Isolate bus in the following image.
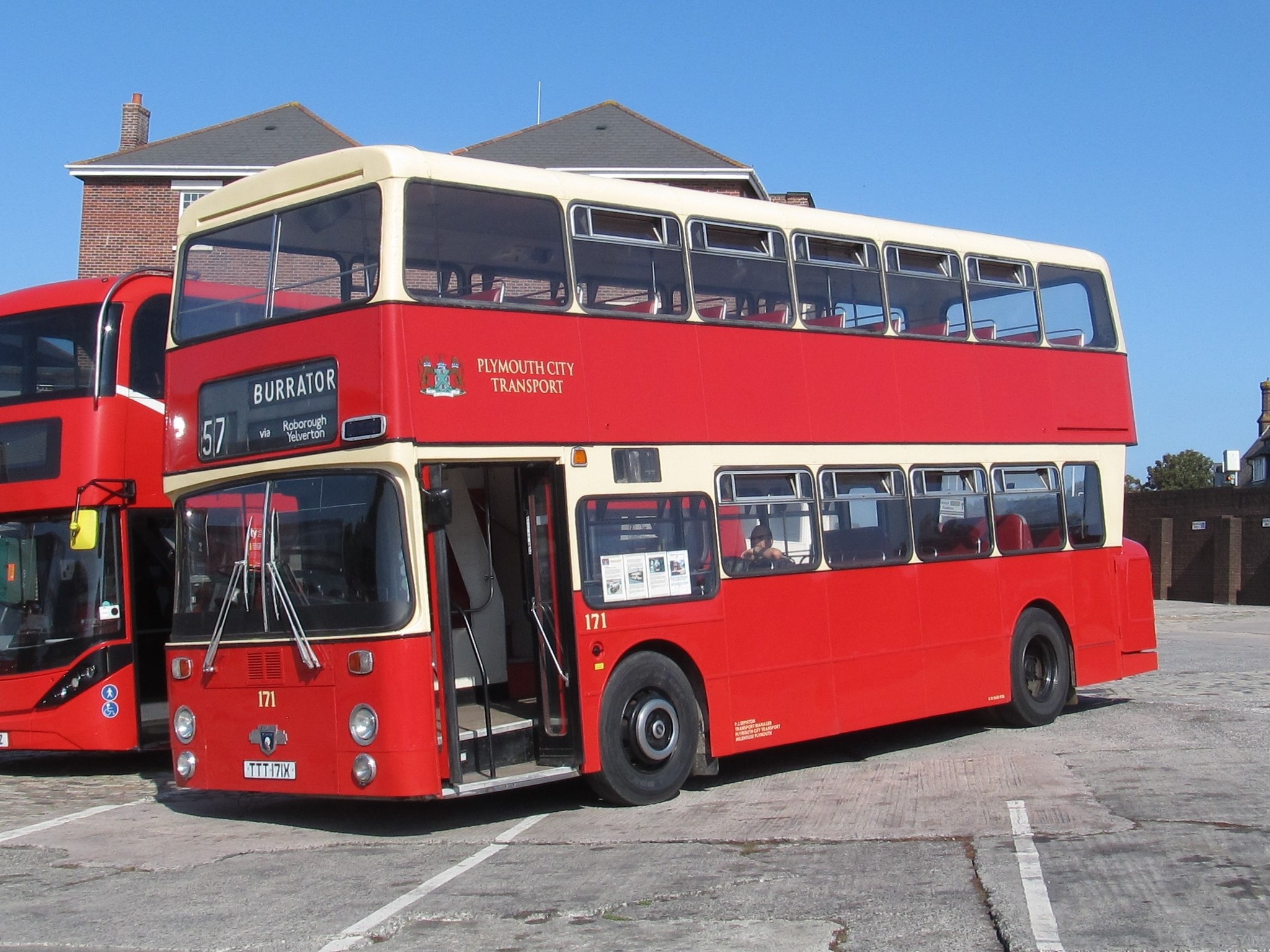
[0,263,341,752]
[160,143,1162,806]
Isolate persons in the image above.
[739,524,785,563]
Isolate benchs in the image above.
[435,277,1086,345]
[823,514,1062,557]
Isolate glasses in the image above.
[749,534,771,542]
